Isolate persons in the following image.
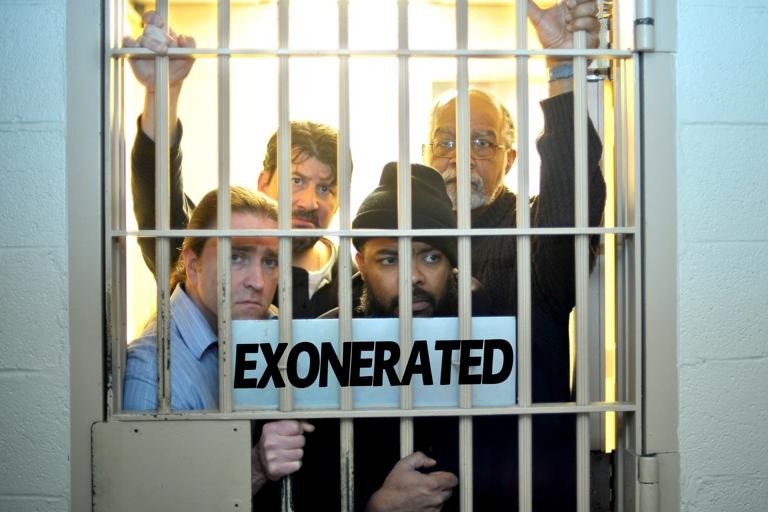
[422,0,605,399]
[122,9,361,319]
[293,161,577,512]
[123,185,315,494]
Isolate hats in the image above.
[352,162,458,267]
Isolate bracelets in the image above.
[548,63,572,82]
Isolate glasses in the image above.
[430,138,505,157]
